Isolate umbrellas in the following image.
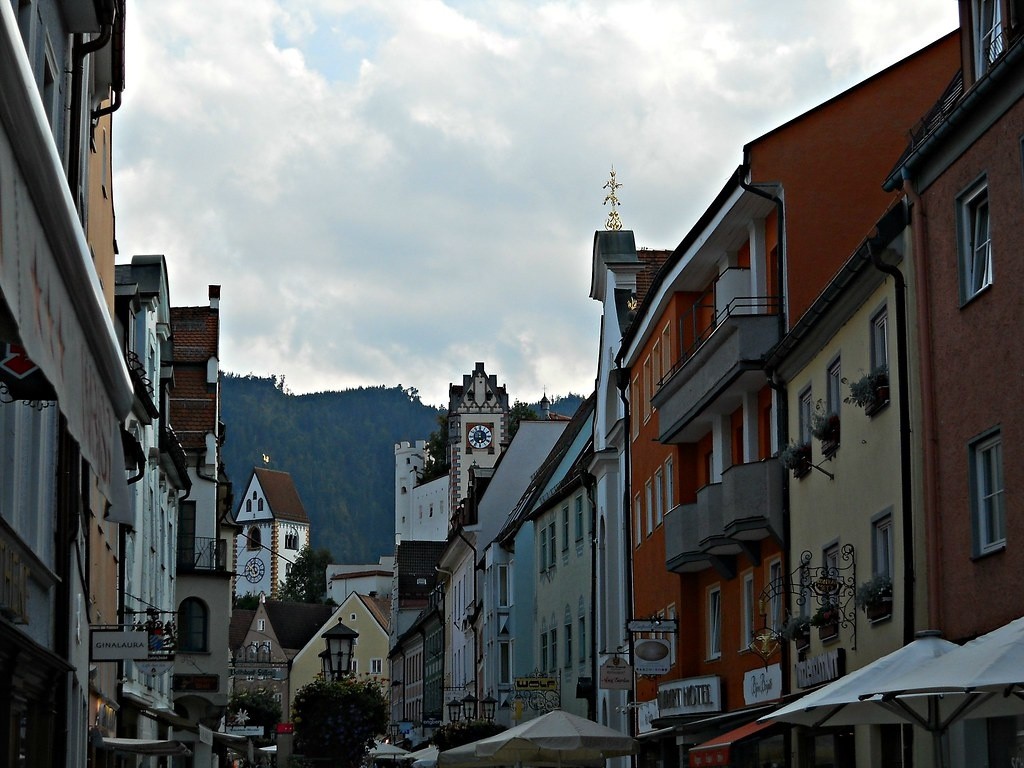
[258,745,277,754]
[758,616,1024,768]
[367,709,641,768]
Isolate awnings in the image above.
[147,707,254,762]
[688,719,780,768]
[102,737,193,759]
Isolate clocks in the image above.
[468,425,492,449]
[245,558,265,583]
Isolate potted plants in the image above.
[803,411,841,455]
[843,363,888,415]
[784,615,811,650]
[854,575,893,619]
[772,438,812,477]
[811,602,840,639]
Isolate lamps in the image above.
[749,543,857,672]
